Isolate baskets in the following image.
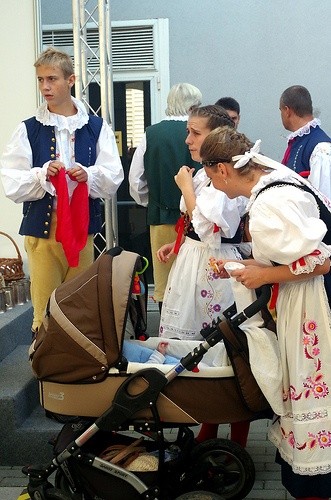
[0,230,24,280]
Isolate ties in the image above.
[281,138,294,164]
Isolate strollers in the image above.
[21,247,284,500]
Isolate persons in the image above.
[0,47,125,423]
[121,82,331,500]
